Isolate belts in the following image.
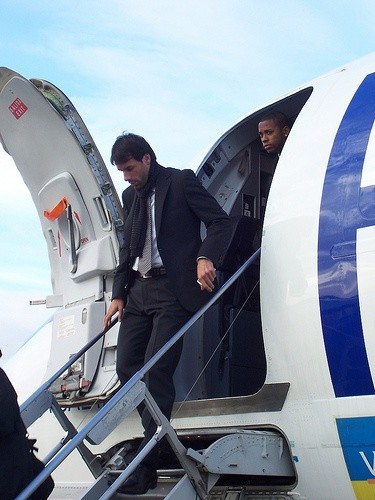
[135,267,167,277]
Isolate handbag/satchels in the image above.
[0,366,54,500]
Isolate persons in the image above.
[103,134,233,495]
[257,111,292,174]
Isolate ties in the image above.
[137,196,151,276]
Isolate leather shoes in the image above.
[107,466,158,495]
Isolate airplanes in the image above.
[0,52,375,500]
